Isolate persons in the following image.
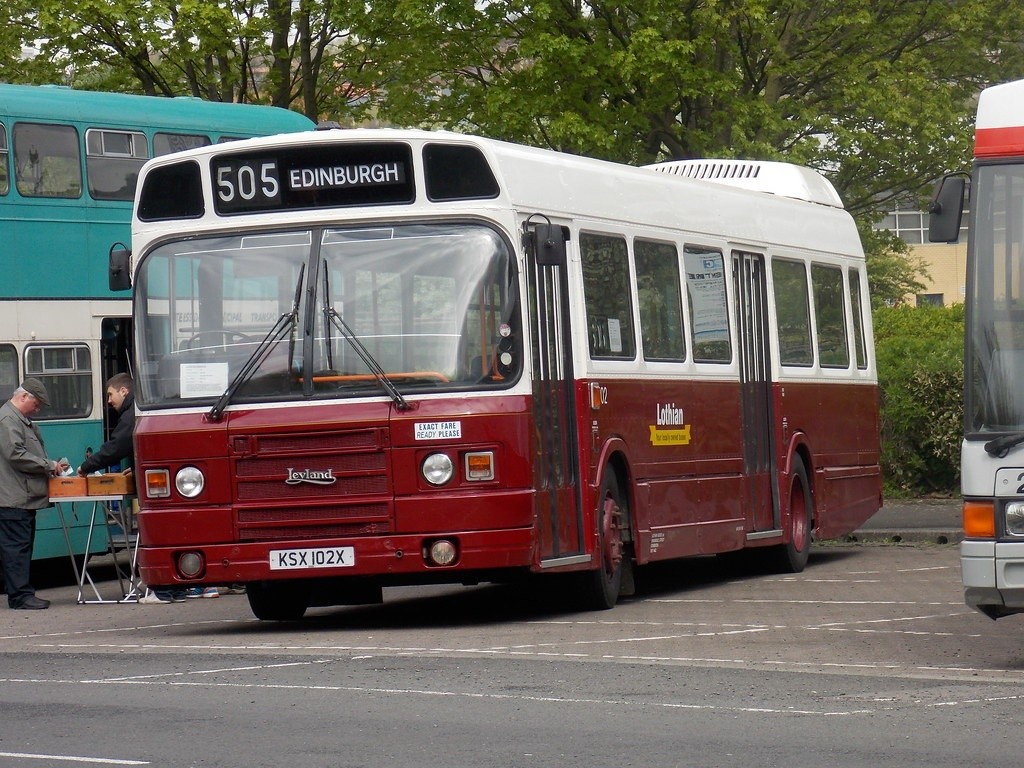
[0,378,67,610]
[186,586,218,597]
[77,373,187,604]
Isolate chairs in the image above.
[228,334,289,379]
[465,341,809,383]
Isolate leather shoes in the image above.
[16,596,50,609]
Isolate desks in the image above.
[49,494,153,604]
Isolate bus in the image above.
[928,77,1024,624]
[106,126,886,622]
[0,85,320,587]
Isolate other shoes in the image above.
[204,587,220,598]
[186,588,203,598]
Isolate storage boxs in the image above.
[87,473,135,495]
[47,476,87,497]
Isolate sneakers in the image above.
[139,591,170,604]
[172,596,186,602]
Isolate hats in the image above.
[21,378,50,406]
